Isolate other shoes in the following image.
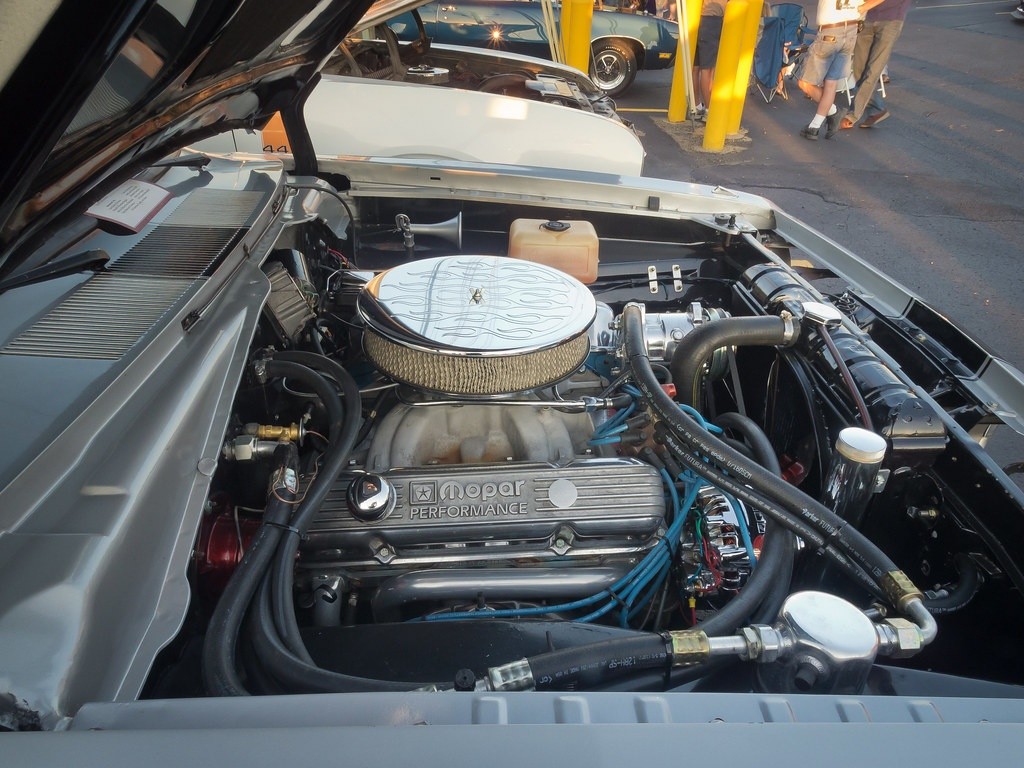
[774,89,783,95]
[840,117,854,128]
[858,108,890,128]
[879,74,889,83]
[687,102,706,120]
[702,110,708,122]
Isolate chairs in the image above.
[750,3,816,103]
[841,53,886,106]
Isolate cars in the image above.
[0,0,1024,768]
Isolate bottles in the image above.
[823,427,886,528]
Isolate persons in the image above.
[840,0,912,129]
[791,0,867,140]
[693,0,728,123]
[656,0,676,21]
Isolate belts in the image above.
[819,20,859,30]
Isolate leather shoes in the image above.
[800,124,819,140]
[825,105,845,139]
